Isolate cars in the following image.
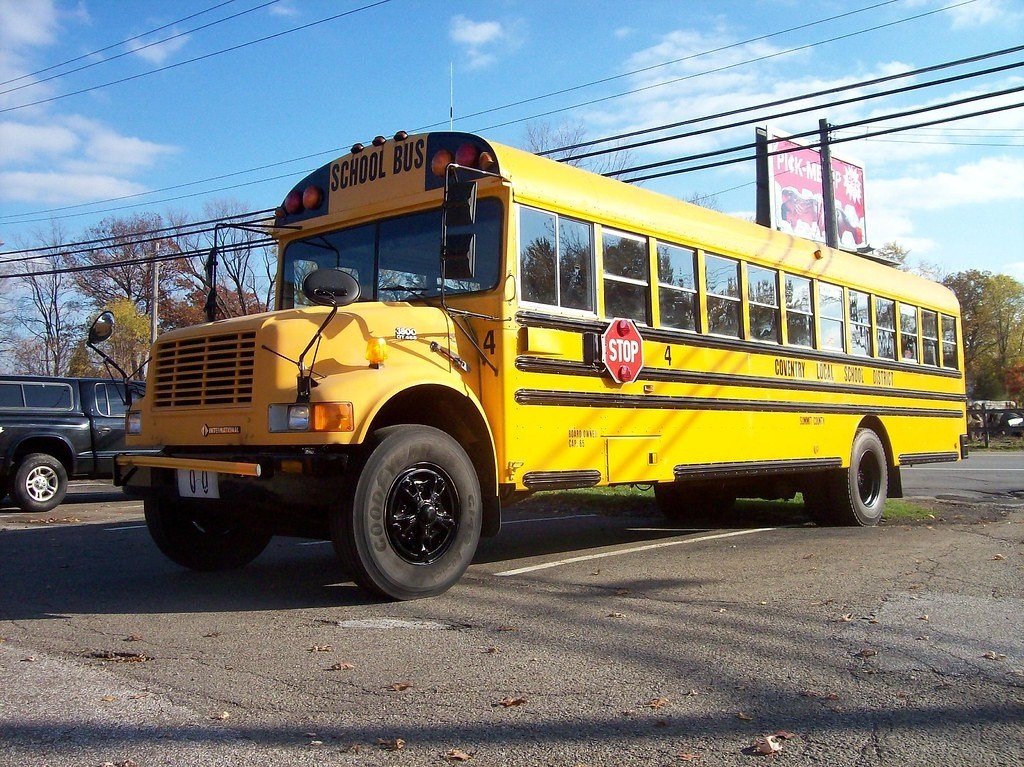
[1000,408,1024,436]
[0,372,148,513]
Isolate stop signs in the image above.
[602,317,644,385]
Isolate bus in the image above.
[972,400,1016,410]
[83,124,970,601]
